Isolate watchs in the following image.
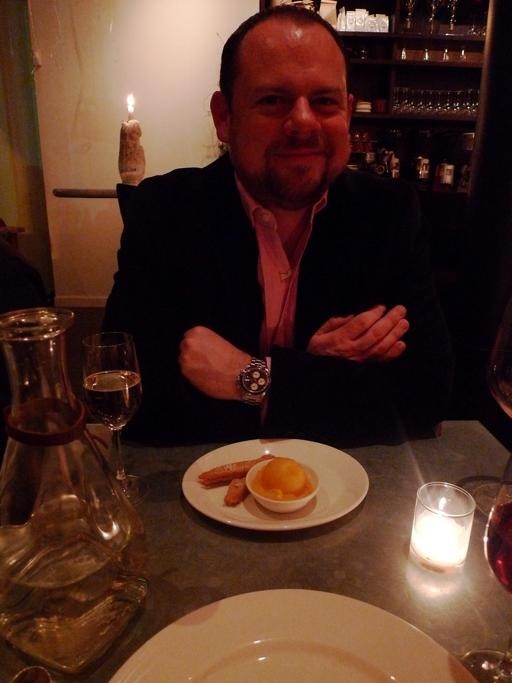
[236,358,272,406]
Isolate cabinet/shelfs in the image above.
[258,0,498,199]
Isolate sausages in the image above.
[198,455,274,506]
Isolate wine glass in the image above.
[394,86,477,116]
[462,304,511,683]
[80,331,154,503]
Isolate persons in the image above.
[100,6,509,449]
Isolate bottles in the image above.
[355,123,458,186]
[0,305,151,673]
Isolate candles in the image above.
[125,94,135,121]
[412,492,472,567]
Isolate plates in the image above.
[107,588,478,683]
[182,436,370,533]
